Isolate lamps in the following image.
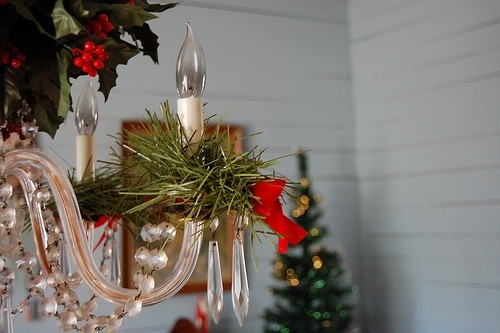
[0,19,312,333]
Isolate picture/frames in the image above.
[120,119,249,295]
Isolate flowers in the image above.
[0,0,184,141]
